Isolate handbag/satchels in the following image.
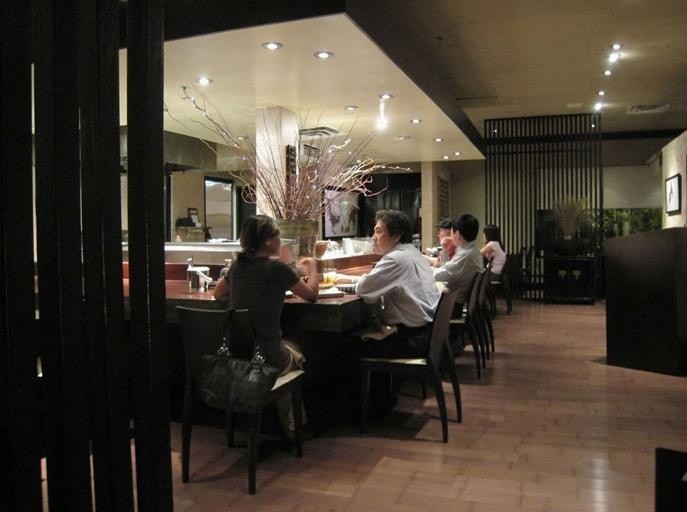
[200,355,279,416]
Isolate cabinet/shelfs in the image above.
[543,255,597,304]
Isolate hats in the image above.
[435,218,452,228]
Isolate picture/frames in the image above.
[322,184,361,237]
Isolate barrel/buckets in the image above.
[187,266,214,293]
[274,219,320,259]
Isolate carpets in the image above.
[655,446,685,508]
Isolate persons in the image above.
[213,215,320,442]
[334,209,445,417]
[480,224,506,282]
[433,214,483,357]
[423,218,456,267]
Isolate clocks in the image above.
[664,173,681,214]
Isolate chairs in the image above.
[175,305,305,495]
[350,248,512,443]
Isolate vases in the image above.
[561,236,573,255]
[275,218,317,256]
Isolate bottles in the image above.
[219,258,234,280]
